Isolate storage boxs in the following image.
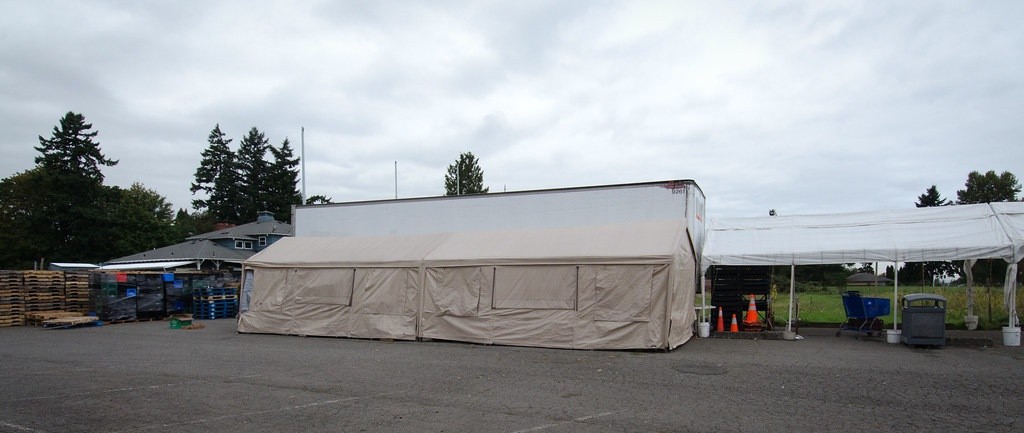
[90,273,193,323]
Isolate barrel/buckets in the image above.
[887,330,902,344]
[964,316,978,330]
[698,322,710,337]
[1003,326,1021,346]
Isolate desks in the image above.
[695,305,716,323]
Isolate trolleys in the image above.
[835,290,890,340]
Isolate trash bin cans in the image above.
[901,293,946,347]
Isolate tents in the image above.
[698,200,1024,332]
[238,218,696,350]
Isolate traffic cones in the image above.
[730,314,739,333]
[742,294,762,323]
[715,306,725,332]
